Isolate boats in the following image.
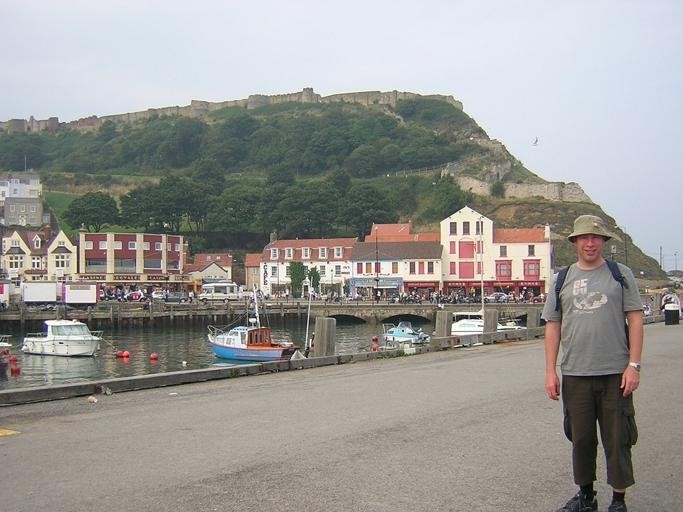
[0,334,12,357]
[20,318,103,359]
[207,282,300,362]
[378,320,429,344]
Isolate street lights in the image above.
[375,226,380,305]
[163,220,170,302]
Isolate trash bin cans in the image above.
[665,304,680,325]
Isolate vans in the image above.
[196,283,239,303]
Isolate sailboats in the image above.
[449,216,526,335]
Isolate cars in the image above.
[390,290,546,304]
[99,289,193,303]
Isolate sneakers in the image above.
[608,503,627,512]
[556,491,597,511]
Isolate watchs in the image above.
[628,362,642,372]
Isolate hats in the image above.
[568,215,611,243]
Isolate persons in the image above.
[98,284,545,304]
[538,214,647,512]
